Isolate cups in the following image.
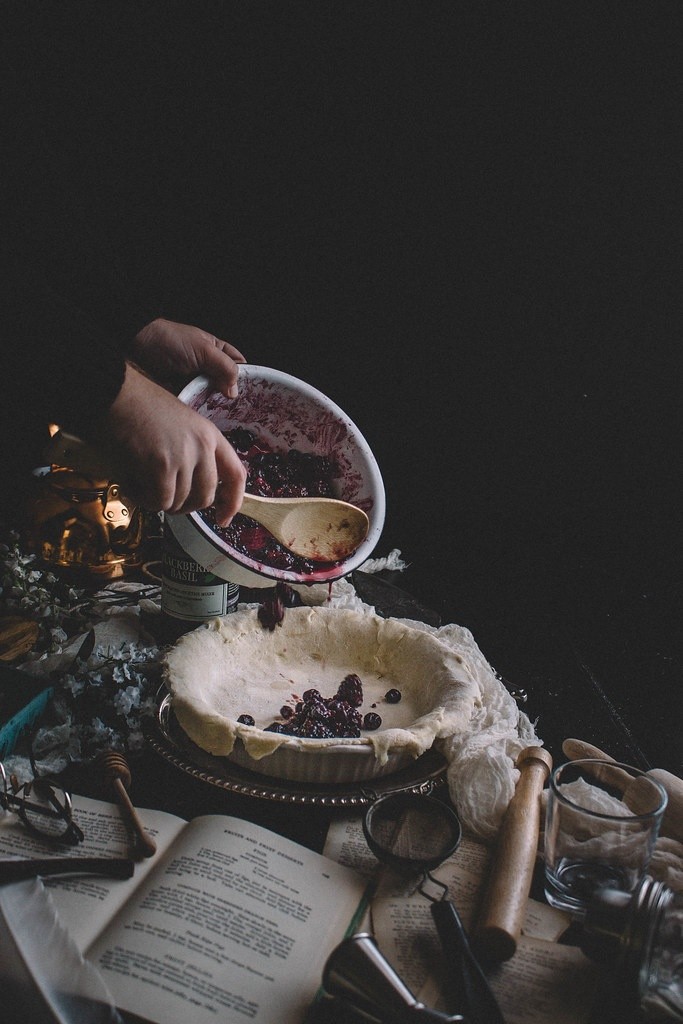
[542,759,668,915]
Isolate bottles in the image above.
[157,534,241,646]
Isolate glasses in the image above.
[0,763,85,842]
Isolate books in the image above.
[0,786,373,1024]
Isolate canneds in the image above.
[160,519,239,634]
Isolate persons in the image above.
[0,255,248,529]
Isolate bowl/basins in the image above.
[160,610,415,781]
[176,374,387,590]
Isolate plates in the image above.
[141,687,449,807]
